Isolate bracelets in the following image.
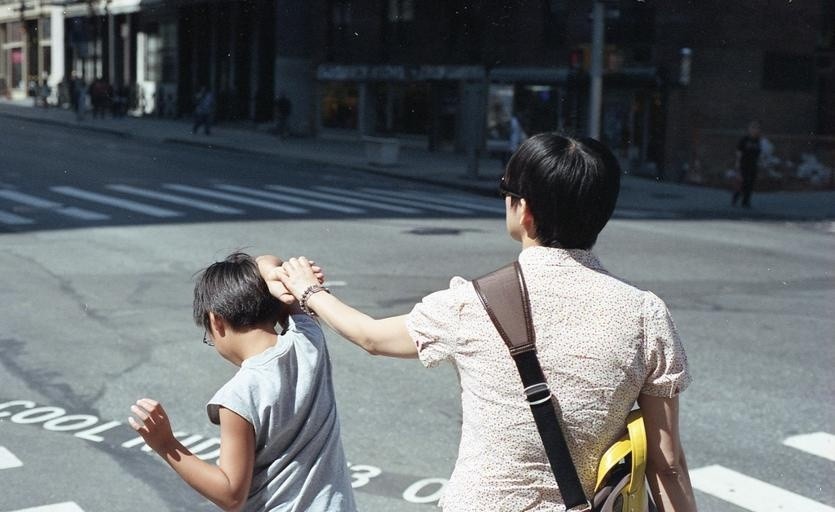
[304,288,330,317]
[299,285,330,316]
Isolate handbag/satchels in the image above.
[591,409,656,512]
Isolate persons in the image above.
[275,131,701,511]
[193,84,216,137]
[487,101,513,168]
[128,248,357,511]
[33,76,136,124]
[731,121,762,211]
[153,79,166,116]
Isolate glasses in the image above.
[203,329,215,347]
[498,176,522,200]
